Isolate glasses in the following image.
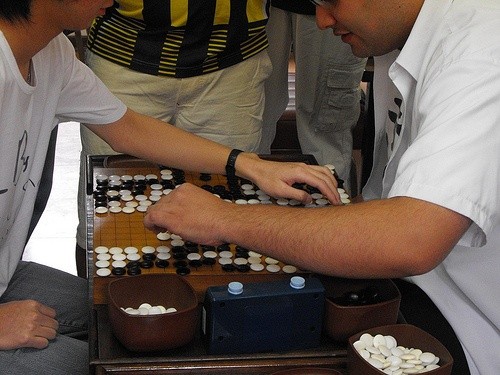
[312,0,340,11]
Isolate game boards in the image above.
[93,164,369,305]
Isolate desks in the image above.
[84,150,397,375]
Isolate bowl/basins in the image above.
[108,273,201,353]
[348,325,453,375]
[323,276,401,344]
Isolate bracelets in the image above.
[225,148,244,177]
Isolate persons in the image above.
[0,0,342,375]
[262,0,368,197]
[75,0,272,279]
[144,0,500,375]
